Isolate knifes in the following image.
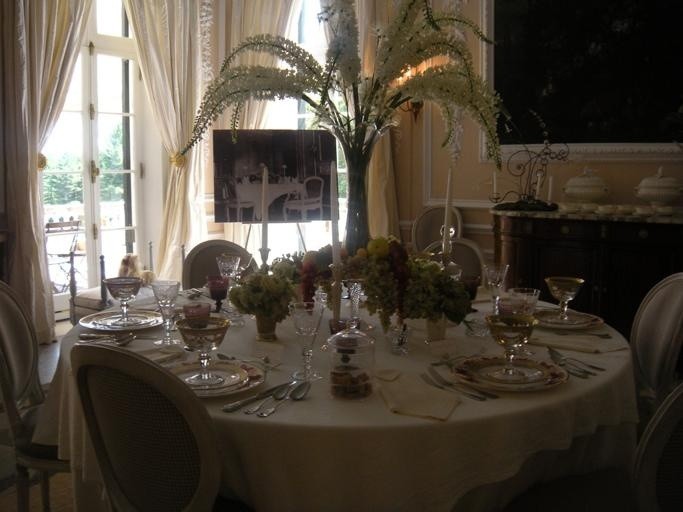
[77,334,158,341]
[544,344,602,379]
[221,382,288,411]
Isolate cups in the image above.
[287,302,326,382]
[391,326,411,357]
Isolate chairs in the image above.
[0,206,683,512]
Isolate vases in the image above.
[337,148,373,260]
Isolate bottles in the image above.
[326,321,376,398]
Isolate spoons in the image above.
[244,383,310,418]
[73,333,135,348]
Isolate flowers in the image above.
[176,1,511,256]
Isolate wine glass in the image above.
[103,253,241,387]
[481,263,582,377]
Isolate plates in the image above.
[78,309,164,332]
[156,360,265,397]
[535,308,605,331]
[452,357,569,390]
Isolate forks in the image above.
[419,366,498,402]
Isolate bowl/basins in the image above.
[557,201,673,216]
